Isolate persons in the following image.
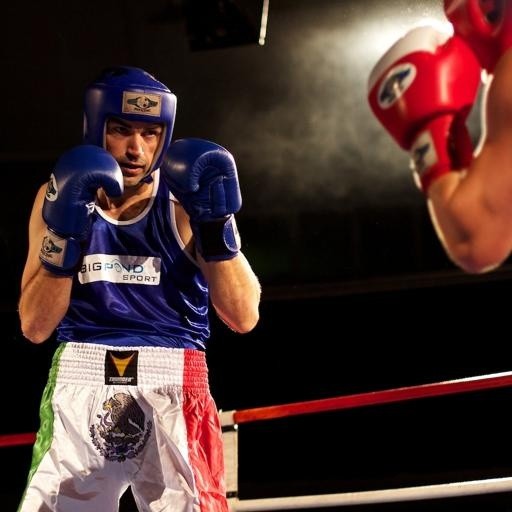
[17,65,265,512]
[362,0,512,275]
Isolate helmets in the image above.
[81,66,178,188]
[442,0,512,76]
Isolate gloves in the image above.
[39,142,128,277]
[165,135,244,262]
[366,25,481,196]
